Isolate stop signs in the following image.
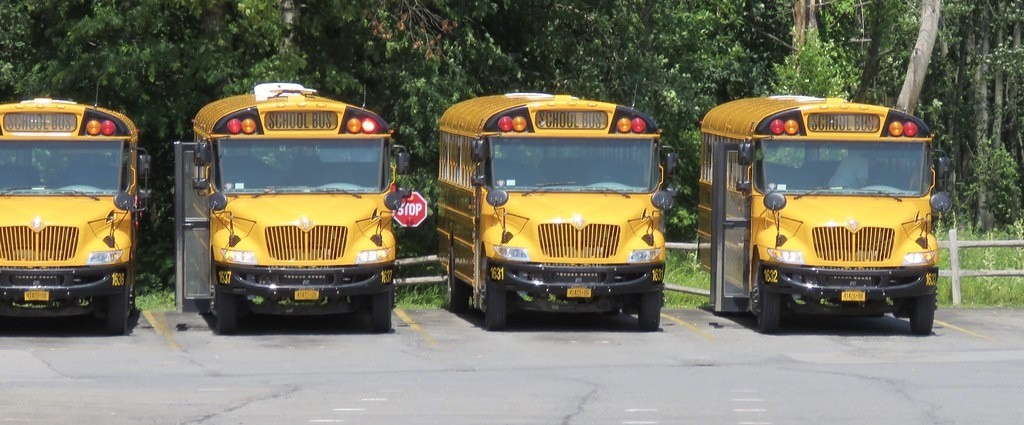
[391,191,428,228]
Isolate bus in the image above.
[0,97,154,334]
[435,92,677,332]
[693,96,953,337]
[174,83,414,335]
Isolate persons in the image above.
[832,150,889,189]
